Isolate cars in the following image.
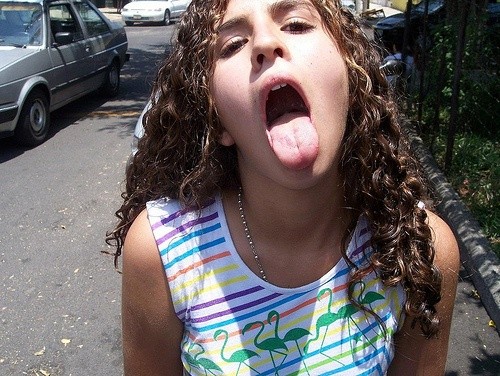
[0,0,130,147]
[120,0,193,26]
[373,1,500,64]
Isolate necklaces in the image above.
[236,187,270,285]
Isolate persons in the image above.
[382,42,416,88]
[100,0,459,376]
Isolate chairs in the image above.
[50,21,65,41]
